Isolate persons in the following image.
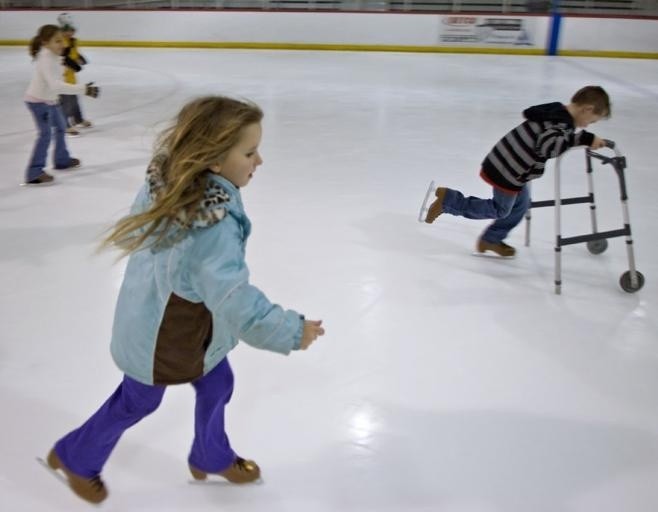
[418,86,610,259]
[23,24,100,185]
[57,26,92,136]
[33,96,325,502]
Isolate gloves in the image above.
[86,82,100,98]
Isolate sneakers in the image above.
[47,447,108,503]
[56,159,80,169]
[27,173,54,183]
[64,120,91,135]
[425,187,447,223]
[477,237,516,256]
[188,456,260,484]
